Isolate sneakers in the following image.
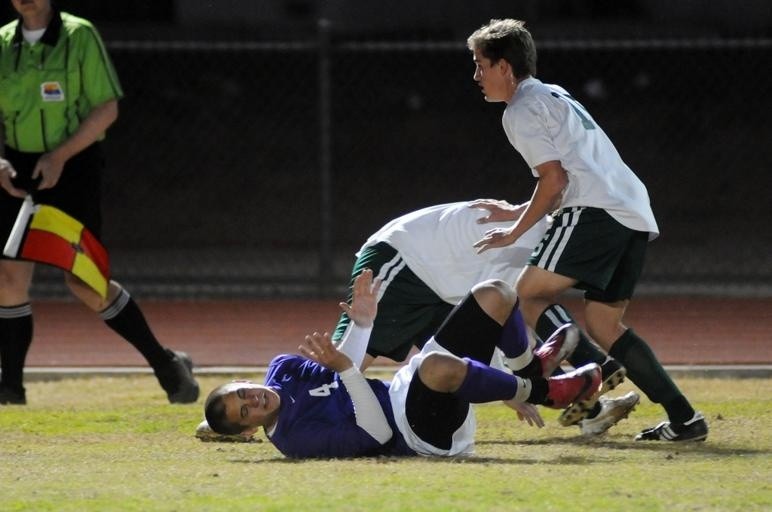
[634,413,710,445]
[159,350,200,402]
[193,421,257,444]
[533,322,641,437]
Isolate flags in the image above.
[4,194,111,300]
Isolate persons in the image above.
[194,198,641,443]
[0,1,202,408]
[204,267,603,460]
[464,16,713,445]
